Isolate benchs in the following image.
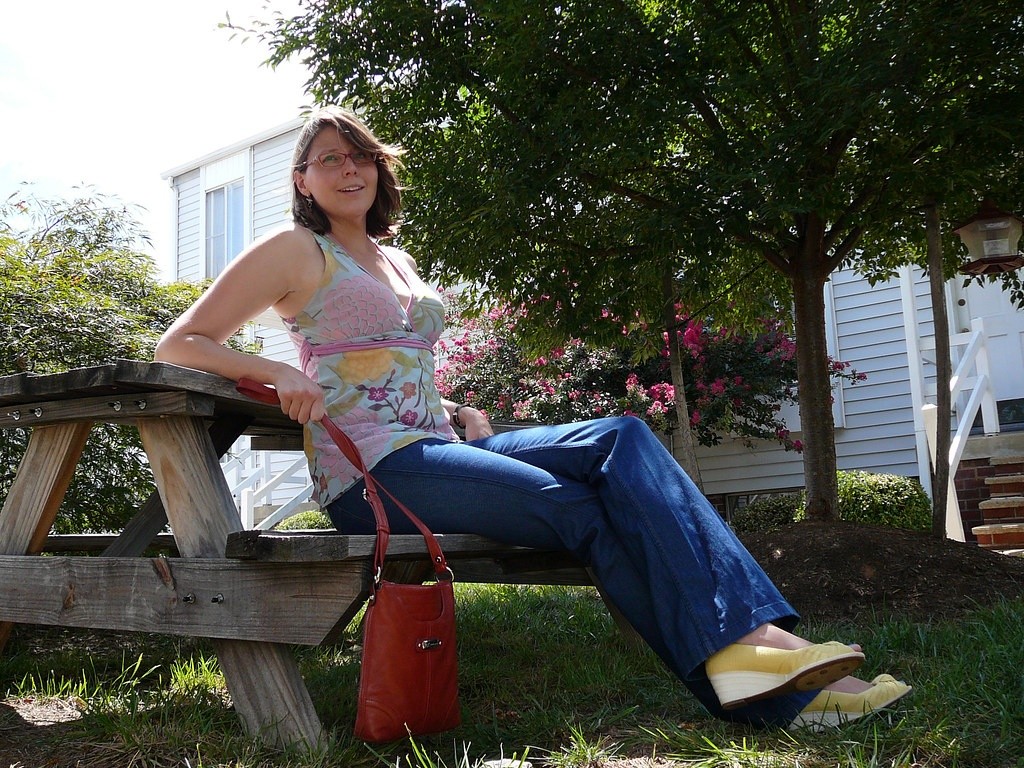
[34,519,262,557]
[212,530,655,557]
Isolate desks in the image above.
[1,358,649,759]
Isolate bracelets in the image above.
[453,404,471,429]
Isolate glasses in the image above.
[300,148,378,172]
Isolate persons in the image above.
[156,105,913,737]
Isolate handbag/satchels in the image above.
[353,576,460,742]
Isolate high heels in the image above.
[706,631,866,712]
[790,672,914,732]
